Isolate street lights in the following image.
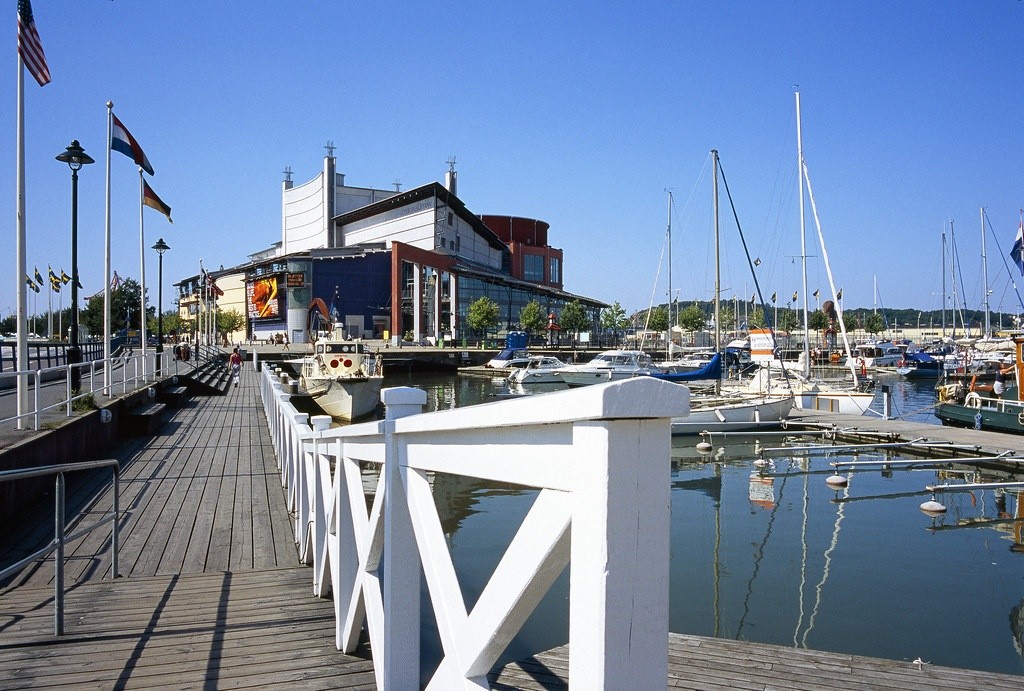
[150,237,171,380]
[55,139,96,404]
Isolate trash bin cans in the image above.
[179,342,188,361]
[438,338,444,349]
[462,339,467,348]
[491,339,498,349]
[482,340,488,350]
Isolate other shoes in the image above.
[235,383,238,387]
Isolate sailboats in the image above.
[669,149,796,434]
[673,205,1024,416]
[935,207,1024,434]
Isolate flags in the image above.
[61,269,71,285]
[35,268,44,286]
[200,263,224,296]
[17,0,52,87]
[48,266,61,293]
[111,113,155,176]
[143,178,173,224]
[26,274,40,293]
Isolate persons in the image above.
[283,333,290,352]
[229,348,244,388]
[268,333,274,341]
[237,345,244,367]
[993,363,1015,407]
[253,334,257,340]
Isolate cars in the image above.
[0,329,115,342]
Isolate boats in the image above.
[282,352,385,379]
[458,331,566,376]
[551,349,669,387]
[508,356,574,384]
[299,286,383,422]
